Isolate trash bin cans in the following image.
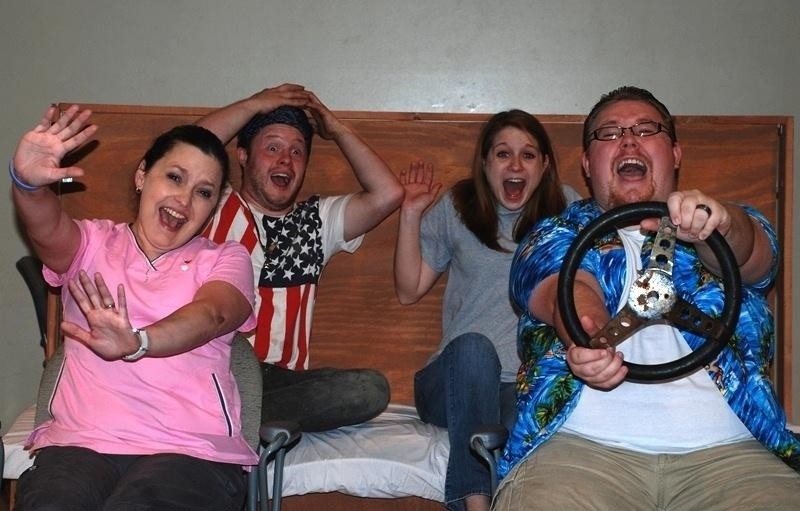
[123,328,150,362]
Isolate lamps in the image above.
[471,423,510,497]
[33,332,301,510]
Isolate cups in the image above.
[588,121,673,141]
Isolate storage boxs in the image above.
[0,103,800,510]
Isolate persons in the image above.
[393,109,584,511]
[490,86,800,511]
[194,84,406,432]
[9,104,259,511]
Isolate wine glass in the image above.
[695,204,712,217]
[105,302,116,310]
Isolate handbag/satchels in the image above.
[9,159,40,194]
